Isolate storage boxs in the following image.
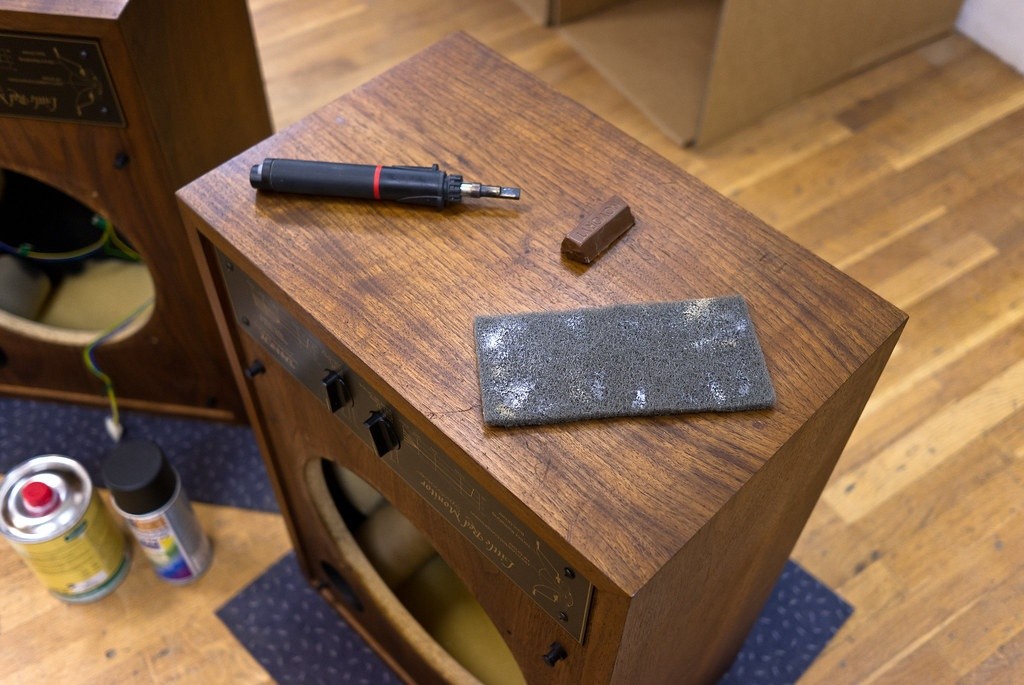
[508,1,966,152]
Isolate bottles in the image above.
[0,453,132,605]
[101,437,214,587]
